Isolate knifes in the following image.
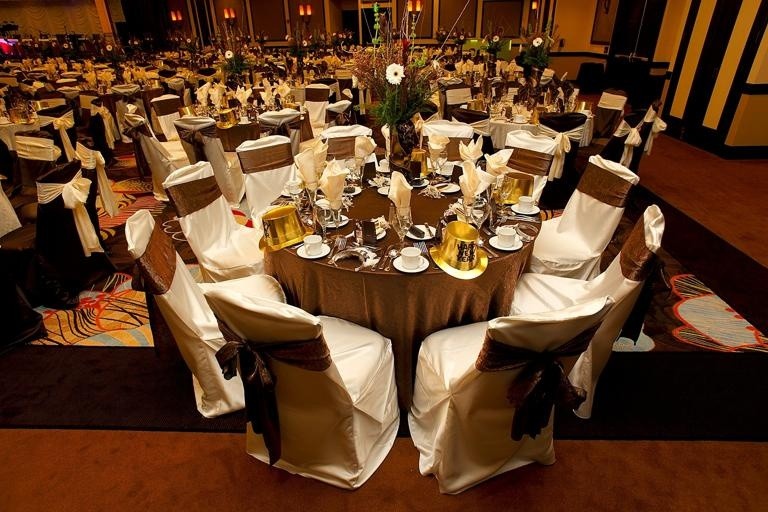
[380,243,394,270]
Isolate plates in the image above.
[280,150,502,245]
[392,256,429,274]
[297,243,330,259]
[511,205,541,216]
[489,237,524,251]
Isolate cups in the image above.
[514,114,524,123]
[304,235,322,255]
[518,195,534,213]
[499,228,516,246]
[401,247,425,268]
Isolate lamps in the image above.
[299,4,312,31]
[171,10,183,31]
[408,0,421,22]
[532,1,538,19]
[224,8,237,27]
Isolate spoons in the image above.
[384,249,399,272]
[328,239,347,265]
[478,239,499,258]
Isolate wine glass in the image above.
[317,152,491,246]
